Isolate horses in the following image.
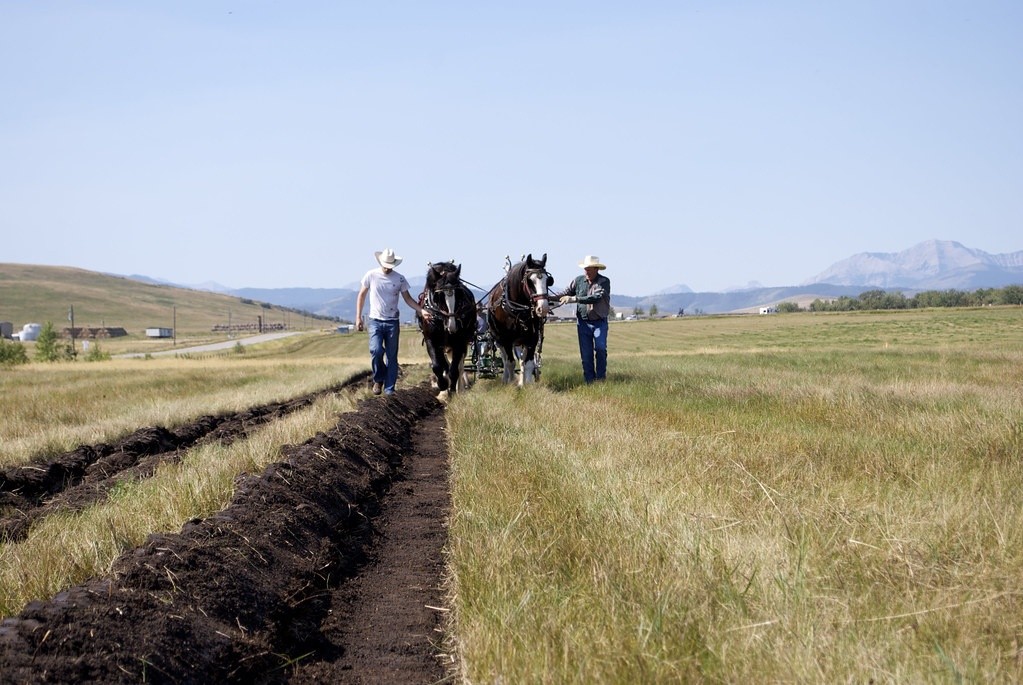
[487,251,550,387]
[418,261,478,403]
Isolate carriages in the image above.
[416,253,555,407]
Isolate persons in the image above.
[476,302,488,333]
[550,255,610,383]
[356,248,434,395]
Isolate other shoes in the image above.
[373,382,382,394]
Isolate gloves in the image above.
[559,295,577,305]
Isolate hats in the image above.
[374,249,403,269]
[476,302,486,310]
[579,256,606,270]
